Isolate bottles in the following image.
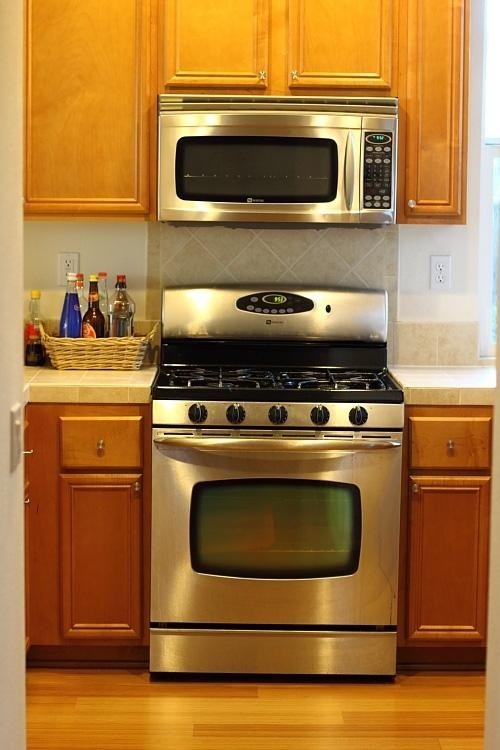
[58,269,134,337]
[22,289,46,362]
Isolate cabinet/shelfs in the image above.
[25,387,150,661]
[24,0,154,219]
[404,387,494,665]
[400,0,470,224]
[157,1,400,92]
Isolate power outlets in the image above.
[57,252,80,288]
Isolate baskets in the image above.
[40,320,158,372]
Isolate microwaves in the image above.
[156,94,400,228]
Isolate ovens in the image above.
[148,427,404,677]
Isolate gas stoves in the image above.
[148,336,406,432]
